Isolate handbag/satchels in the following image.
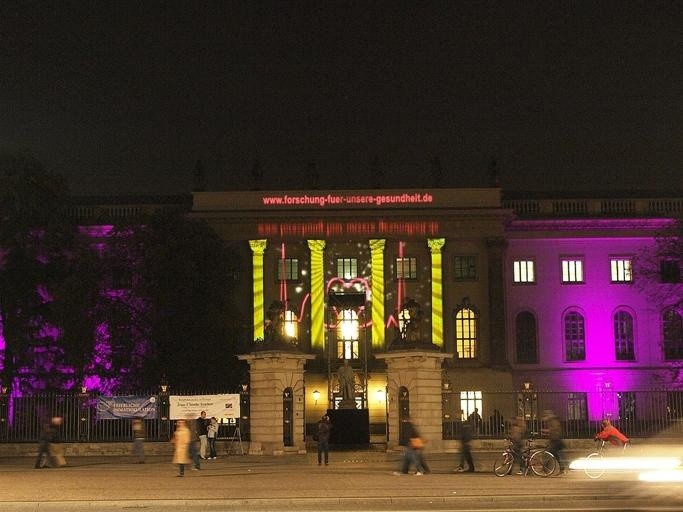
[313,434,319,441]
[409,438,424,450]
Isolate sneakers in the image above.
[318,463,329,465]
[390,470,424,476]
[175,468,200,477]
[34,463,67,468]
[201,456,217,460]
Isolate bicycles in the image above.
[493,437,638,479]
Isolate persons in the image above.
[311,415,332,465]
[48,414,68,466]
[393,403,569,477]
[33,423,57,469]
[594,419,629,454]
[166,407,218,476]
[126,419,144,464]
